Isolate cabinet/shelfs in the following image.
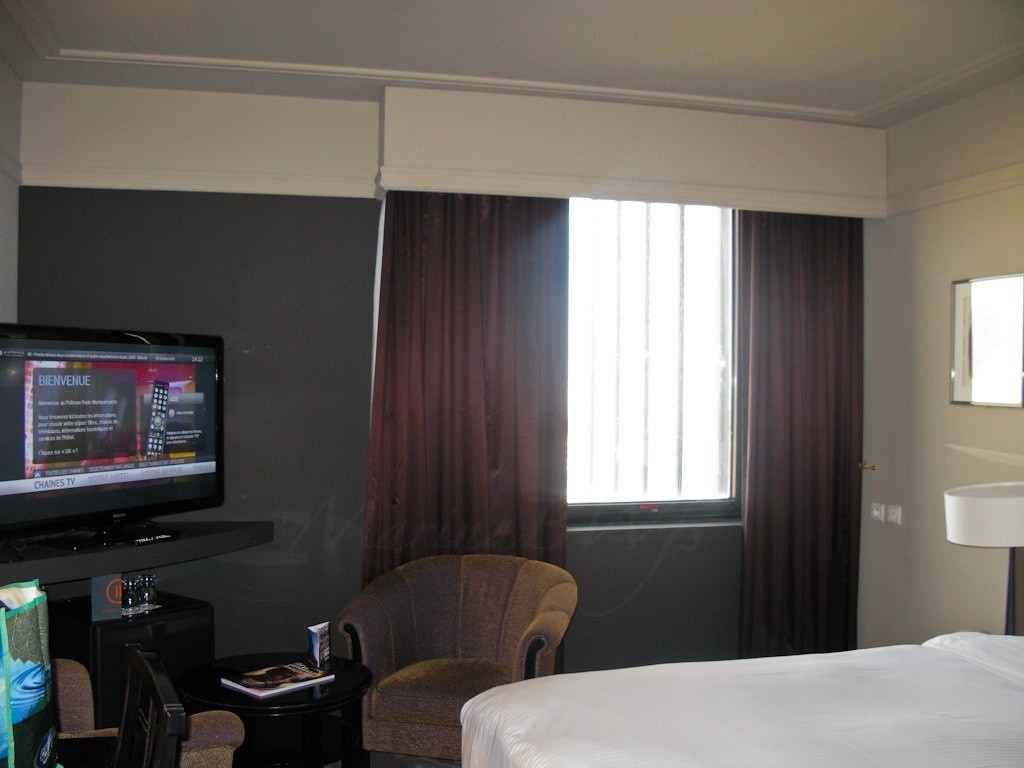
[1,519,274,729]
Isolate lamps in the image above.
[945,482,1024,637]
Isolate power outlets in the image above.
[887,503,902,525]
[870,503,885,521]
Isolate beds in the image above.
[460,634,1024,767]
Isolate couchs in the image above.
[47,654,244,768]
[335,555,578,762]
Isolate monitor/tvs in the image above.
[0,322,226,541]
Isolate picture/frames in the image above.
[947,273,1024,411]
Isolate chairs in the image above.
[114,652,189,768]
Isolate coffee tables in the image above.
[200,651,376,767]
